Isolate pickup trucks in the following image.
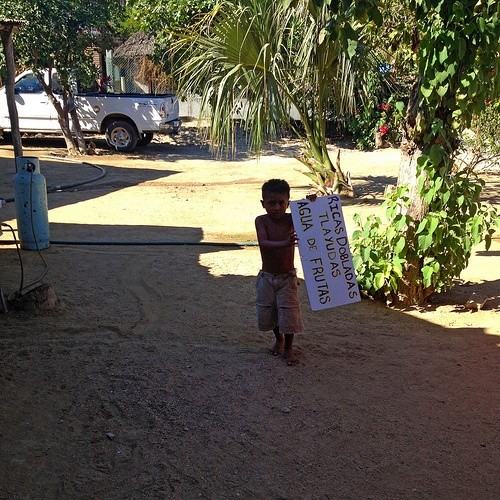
[0,65,182,152]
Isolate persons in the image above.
[254,178,318,366]
